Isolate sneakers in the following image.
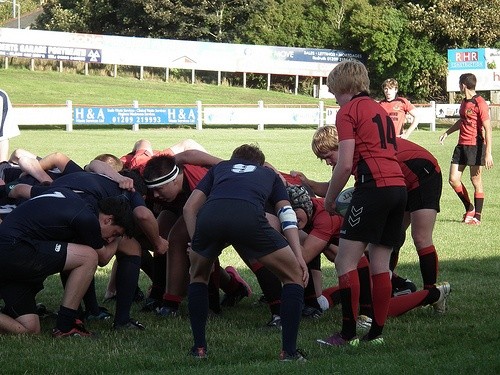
[102,296,116,304]
[112,318,145,329]
[355,315,373,335]
[225,266,251,300]
[302,308,323,319]
[349,334,384,347]
[134,288,144,302]
[391,279,417,298]
[471,218,480,224]
[191,346,208,359]
[51,319,92,337]
[316,333,358,346]
[85,306,113,321]
[139,298,160,313]
[267,315,282,331]
[155,306,178,322]
[431,281,452,315]
[279,349,308,361]
[464,207,476,223]
[36,303,47,316]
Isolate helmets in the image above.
[286,184,313,225]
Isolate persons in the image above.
[317,60,407,347]
[0,139,451,338]
[290,125,443,308]
[378,78,420,140]
[183,143,313,362]
[0,89,21,162]
[440,73,493,225]
